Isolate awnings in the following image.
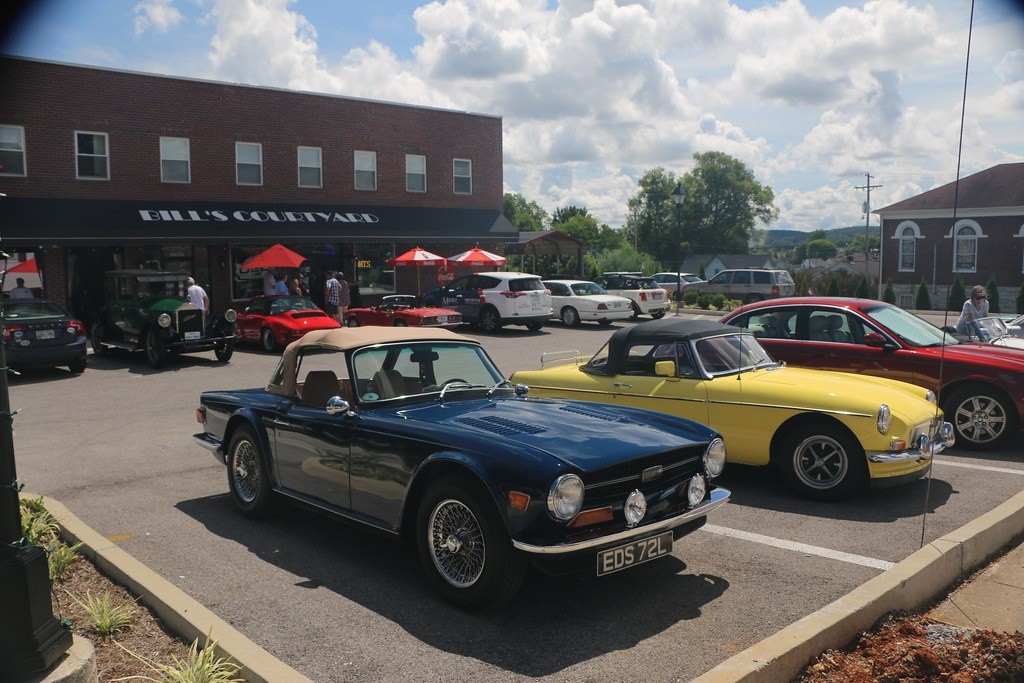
[0,200,520,248]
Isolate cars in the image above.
[639,272,704,302]
[661,296,1024,454]
[540,280,635,329]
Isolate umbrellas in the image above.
[447,248,506,272]
[241,244,306,271]
[388,248,447,295]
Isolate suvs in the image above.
[681,267,797,306]
[415,271,555,336]
[577,272,670,319]
[84,269,239,372]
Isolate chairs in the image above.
[373,370,408,401]
[758,314,848,343]
[303,370,342,406]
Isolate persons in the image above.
[264,269,310,296]
[10,278,32,298]
[326,272,350,323]
[954,285,991,334]
[188,278,209,314]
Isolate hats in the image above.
[335,272,344,278]
[297,273,305,278]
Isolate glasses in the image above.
[974,295,987,300]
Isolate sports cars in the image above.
[343,294,464,332]
[506,317,957,505]
[232,295,342,353]
[0,298,89,374]
[192,326,734,607]
